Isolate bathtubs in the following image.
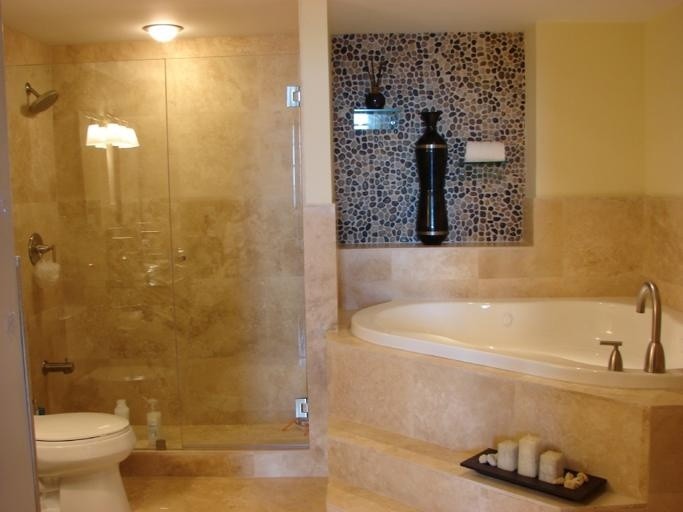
[349,296,683,389]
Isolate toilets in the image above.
[32,411,135,512]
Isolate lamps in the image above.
[141,22,184,42]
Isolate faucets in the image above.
[636,281,661,341]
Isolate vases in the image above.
[414,111,449,244]
